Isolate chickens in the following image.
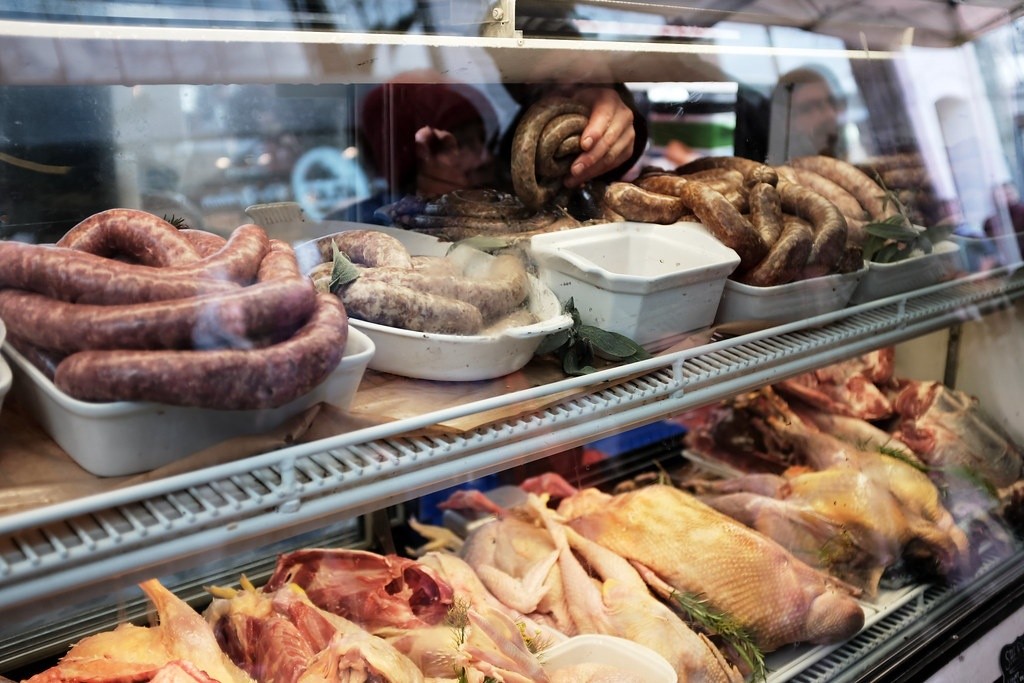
[24,384,975,682]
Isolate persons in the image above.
[763,66,849,165]
[167,0,651,239]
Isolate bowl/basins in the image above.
[527,220,742,357]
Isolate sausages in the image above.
[594,155,917,288]
[374,96,592,243]
[0,209,346,409]
[309,230,529,335]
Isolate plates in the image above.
[848,237,967,307]
[711,261,871,344]
[941,229,1023,280]
[279,228,581,385]
[0,217,379,479]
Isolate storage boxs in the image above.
[0,2,1021,683]
